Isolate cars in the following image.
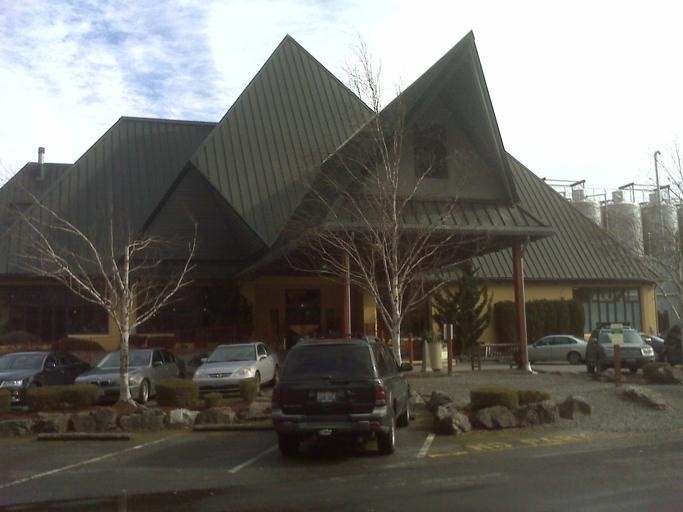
[71,346,189,406]
[189,338,279,401]
[638,333,678,365]
[510,333,590,368]
[0,350,95,407]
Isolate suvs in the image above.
[663,322,682,368]
[579,326,657,375]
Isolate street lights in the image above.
[653,151,666,257]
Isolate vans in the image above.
[270,334,417,458]
